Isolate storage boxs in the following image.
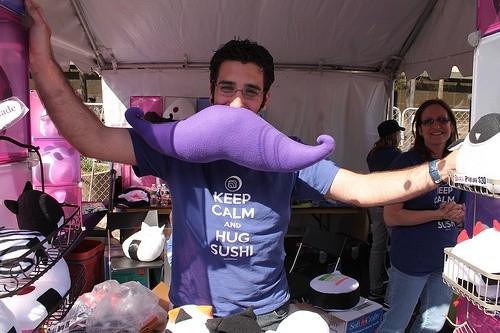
[328,296,383,333]
[105,244,150,289]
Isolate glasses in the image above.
[213,81,263,100]
[421,117,450,127]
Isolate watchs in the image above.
[425,156,446,188]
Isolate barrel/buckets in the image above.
[63,239,106,295]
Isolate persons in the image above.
[365,119,408,310]
[21,0,466,333]
[377,94,467,333]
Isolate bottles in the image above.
[159,183,169,207]
[150,184,158,207]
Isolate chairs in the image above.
[286,224,348,305]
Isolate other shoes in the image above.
[383,299,417,315]
[368,288,386,300]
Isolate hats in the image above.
[377,119,407,137]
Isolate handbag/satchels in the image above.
[115,187,152,209]
